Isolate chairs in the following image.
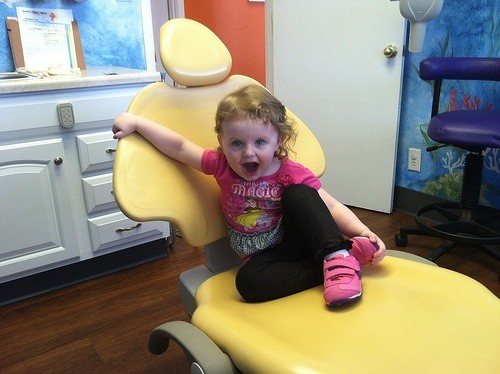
[112,17,500,374]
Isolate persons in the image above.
[113,84,385,308]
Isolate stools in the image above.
[395,56,500,262]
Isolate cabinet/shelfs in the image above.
[0,65,174,309]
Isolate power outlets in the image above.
[408,148,421,172]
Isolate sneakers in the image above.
[340,236,379,269]
[323,248,362,309]
